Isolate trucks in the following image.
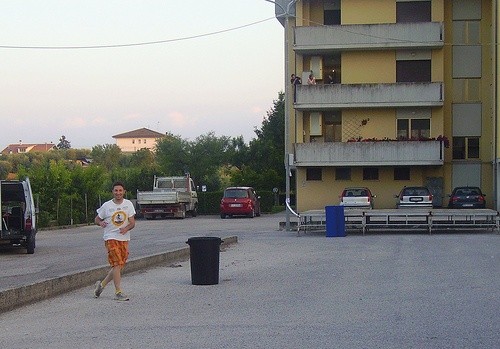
[137,175,207,221]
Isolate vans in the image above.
[220,187,262,218]
[1,179,36,254]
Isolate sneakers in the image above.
[114,292,130,301]
[94,280,104,297]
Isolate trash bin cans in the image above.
[325,205,345,237]
[185,237,224,285]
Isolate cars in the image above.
[393,185,434,208]
[339,187,376,210]
[447,187,487,208]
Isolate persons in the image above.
[94,182,136,301]
[291,74,302,102]
[328,75,336,84]
[307,75,316,85]
